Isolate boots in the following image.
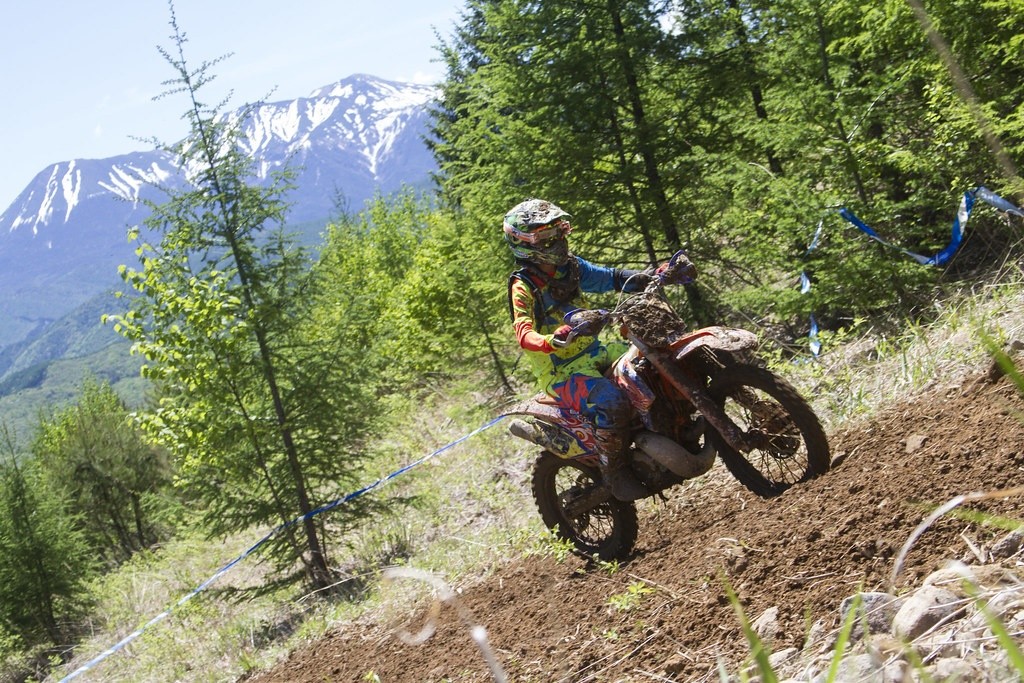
[594,428,644,501]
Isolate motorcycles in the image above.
[503,249,832,562]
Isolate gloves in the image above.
[656,254,698,283]
[553,325,573,348]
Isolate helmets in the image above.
[503,200,572,265]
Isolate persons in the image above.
[503,199,697,502]
